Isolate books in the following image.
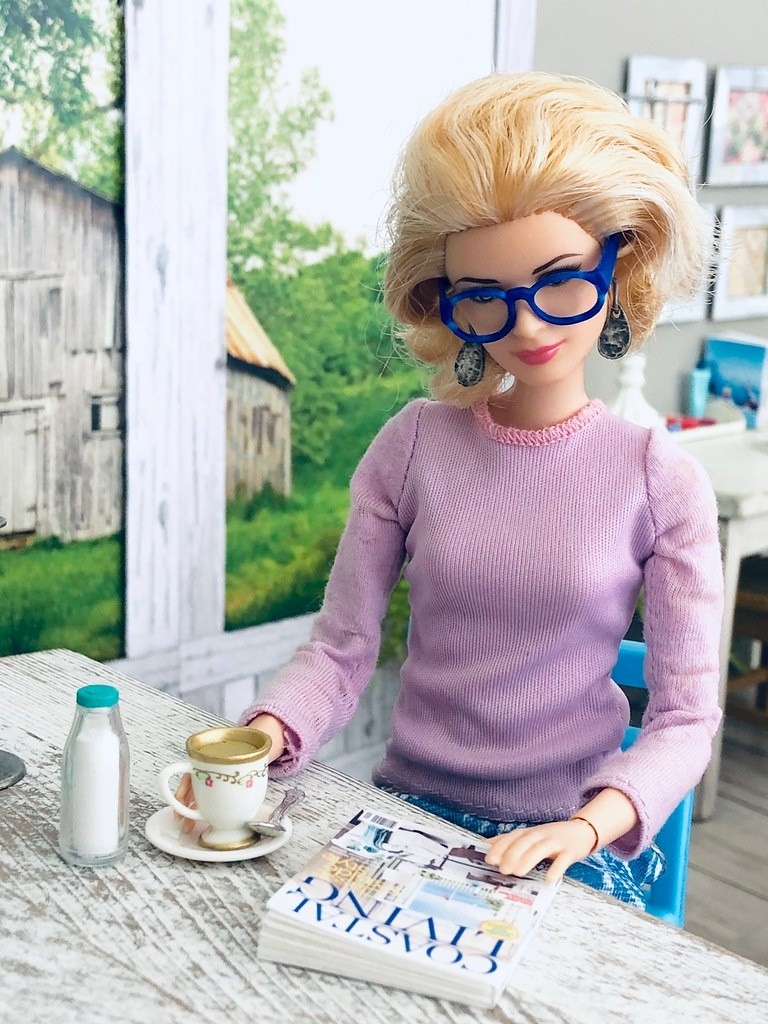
[256,805,563,1009]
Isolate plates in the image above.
[144,803,294,862]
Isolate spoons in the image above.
[248,789,305,837]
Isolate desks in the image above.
[0,646,768,1022]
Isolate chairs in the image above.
[566,636,690,925]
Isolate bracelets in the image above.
[567,812,608,856]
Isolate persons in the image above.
[232,67,722,913]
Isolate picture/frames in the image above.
[709,66,768,191]
[710,202,768,322]
[626,58,711,191]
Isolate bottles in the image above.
[55,684,131,867]
[687,358,713,419]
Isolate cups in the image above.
[158,727,273,851]
